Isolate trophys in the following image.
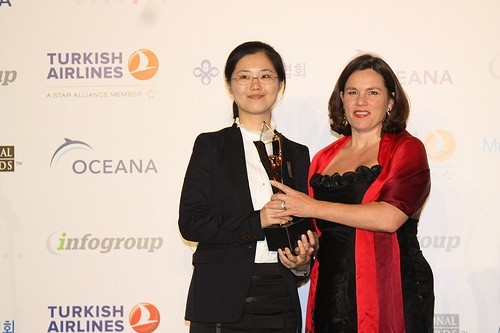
[259,121,309,251]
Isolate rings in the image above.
[281,201,286,208]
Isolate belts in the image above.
[254,262,282,276]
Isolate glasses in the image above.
[231,73,278,85]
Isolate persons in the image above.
[178,40,314,333]
[263,55,435,333]
